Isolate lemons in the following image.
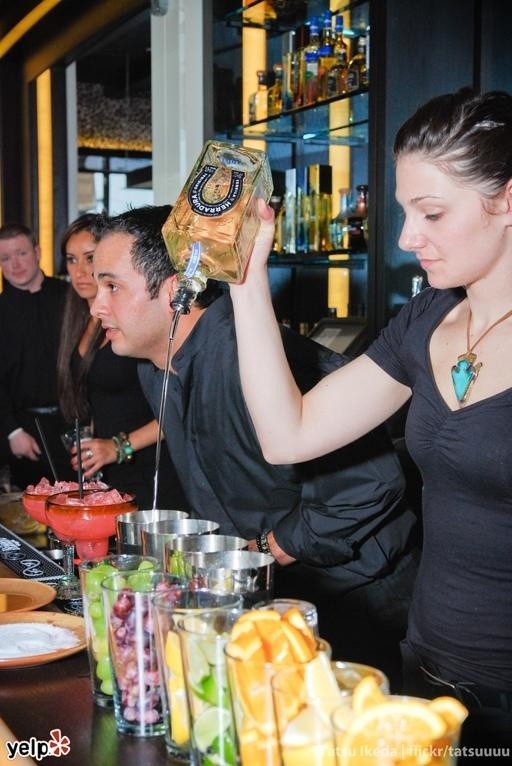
[167,614,241,766]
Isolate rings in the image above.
[85,450,91,459]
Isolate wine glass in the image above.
[24,491,82,590]
[46,488,139,614]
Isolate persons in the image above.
[53,212,193,519]
[1,221,83,493]
[89,203,421,699]
[226,83,512,766]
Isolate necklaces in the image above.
[450,305,512,402]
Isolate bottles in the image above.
[160,141,276,343]
[249,12,369,259]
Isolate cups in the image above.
[78,510,465,765]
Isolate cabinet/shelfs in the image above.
[217,0,367,267]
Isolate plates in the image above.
[1,577,58,614]
[1,611,88,668]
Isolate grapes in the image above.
[82,560,181,725]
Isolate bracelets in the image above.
[254,528,284,575]
[112,435,123,465]
[118,432,133,463]
